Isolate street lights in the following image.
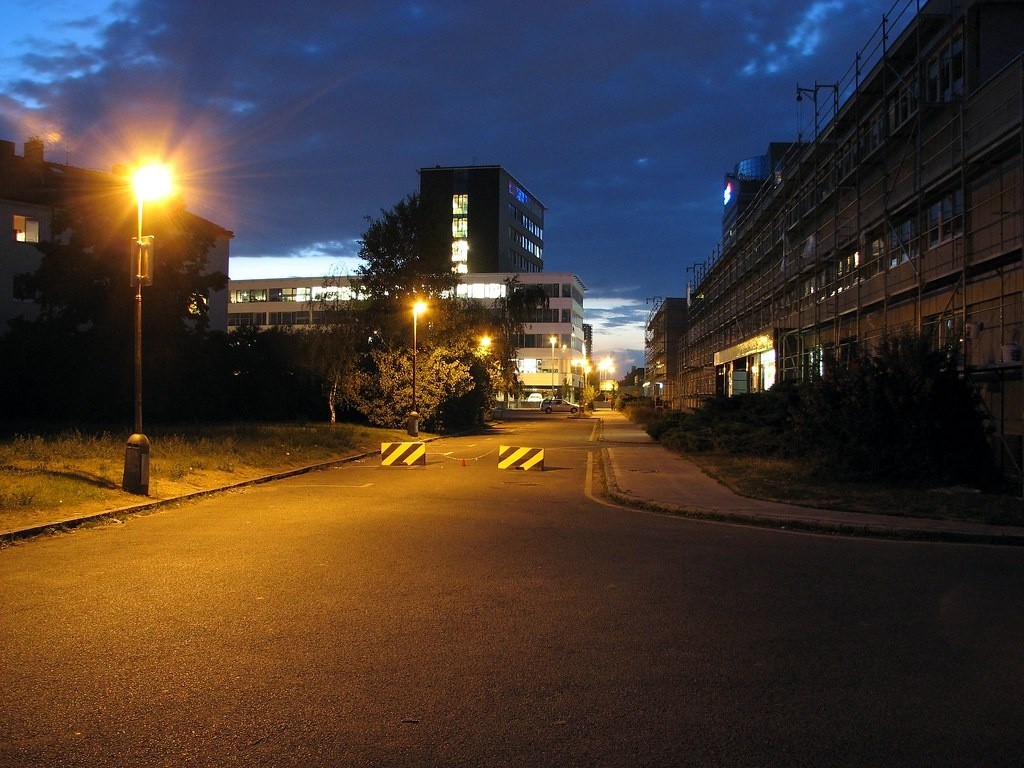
[404,299,430,436]
[580,359,616,396]
[116,153,185,491]
[549,334,557,396]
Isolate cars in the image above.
[541,399,581,413]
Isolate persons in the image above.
[611,399,615,410]
